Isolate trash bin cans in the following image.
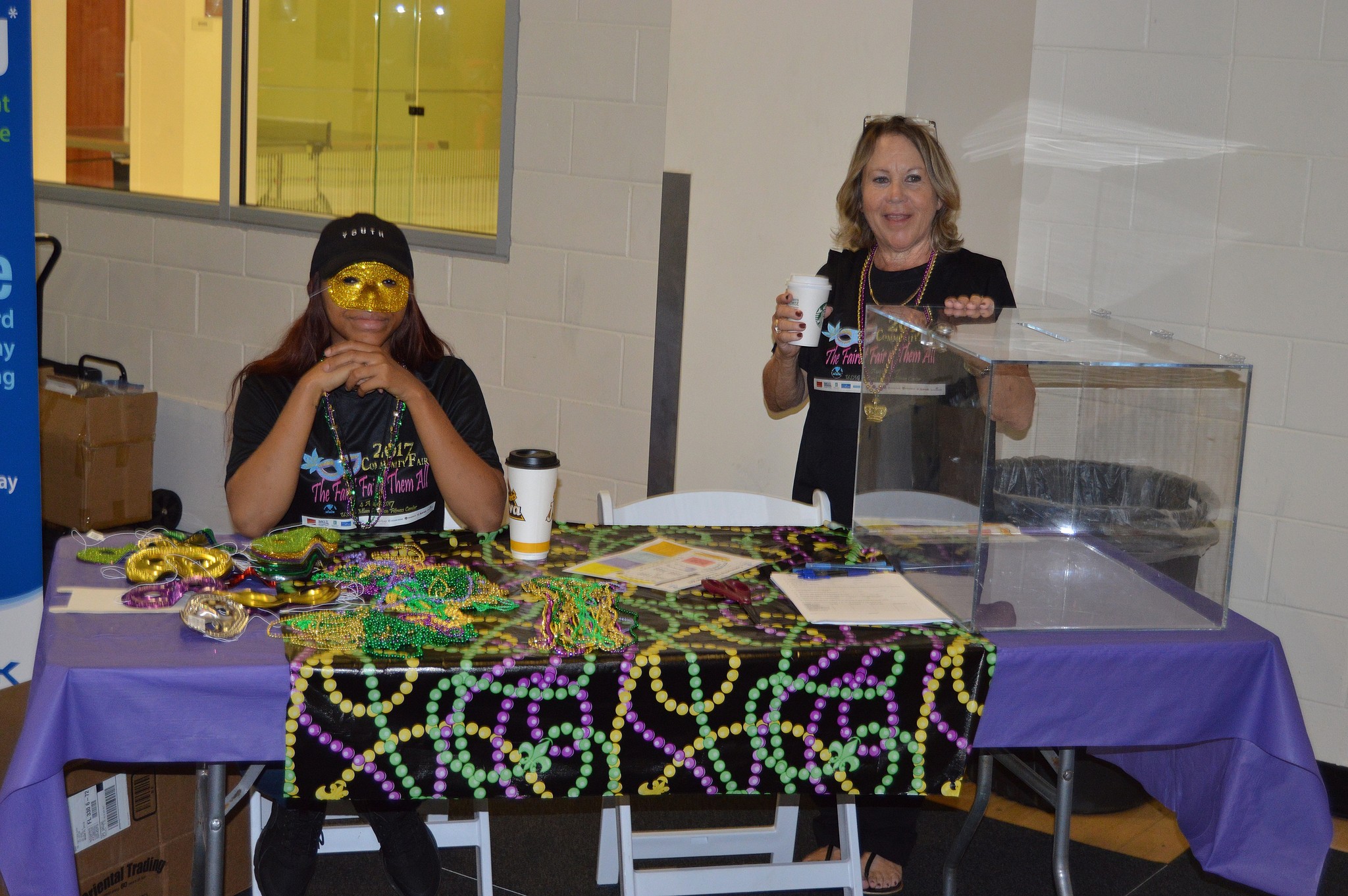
[114,158,130,190]
[993,459,1219,591]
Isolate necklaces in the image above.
[126,543,638,656]
[858,244,939,422]
[319,352,407,529]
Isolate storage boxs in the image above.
[847,288,1259,630]
[35,353,250,896]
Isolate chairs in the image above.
[592,481,866,896]
[245,499,496,896]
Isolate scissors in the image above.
[701,579,761,625]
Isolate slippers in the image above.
[799,839,842,862]
[859,850,907,896]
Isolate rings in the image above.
[774,319,784,334]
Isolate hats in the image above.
[309,212,413,278]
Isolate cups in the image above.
[504,448,562,560]
[786,275,833,347]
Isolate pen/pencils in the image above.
[792,562,886,572]
[806,563,894,572]
[797,569,879,580]
[900,561,975,576]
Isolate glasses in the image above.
[862,115,939,147]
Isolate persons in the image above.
[224,212,506,538]
[763,119,1036,894]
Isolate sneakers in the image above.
[253,803,326,896]
[359,803,442,896]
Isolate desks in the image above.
[0,524,1336,896]
